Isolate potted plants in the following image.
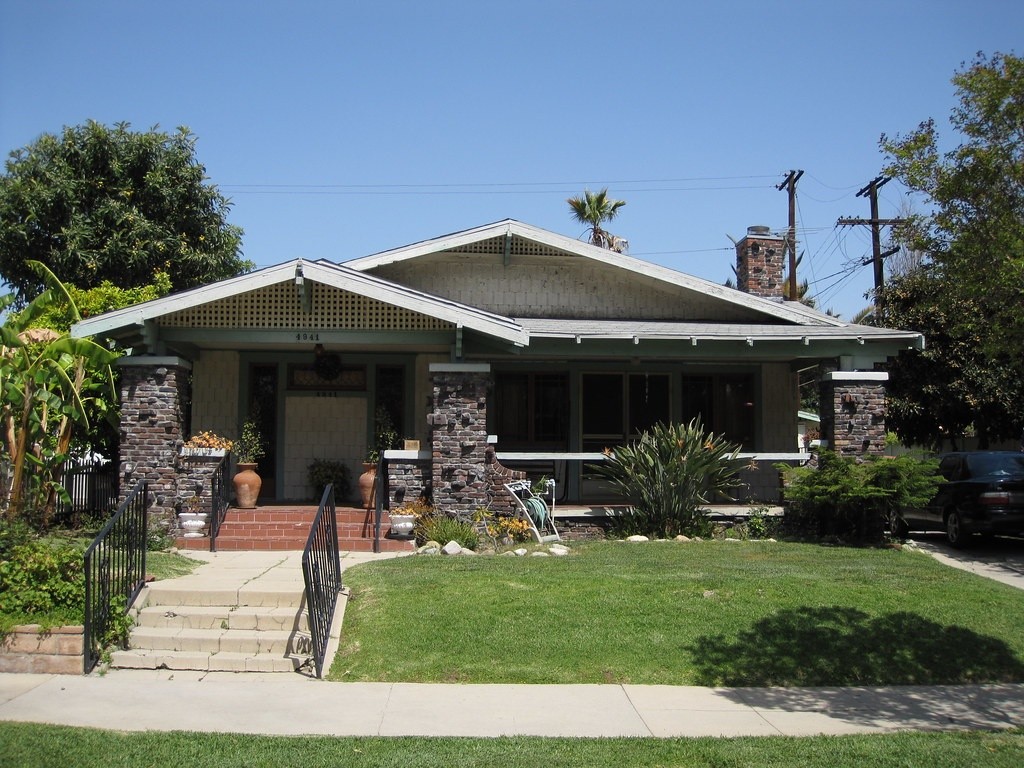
[358,405,411,508]
[389,507,422,535]
[231,422,270,507]
[178,495,208,537]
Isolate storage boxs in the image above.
[405,440,421,450]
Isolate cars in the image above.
[888,449,1023,547]
